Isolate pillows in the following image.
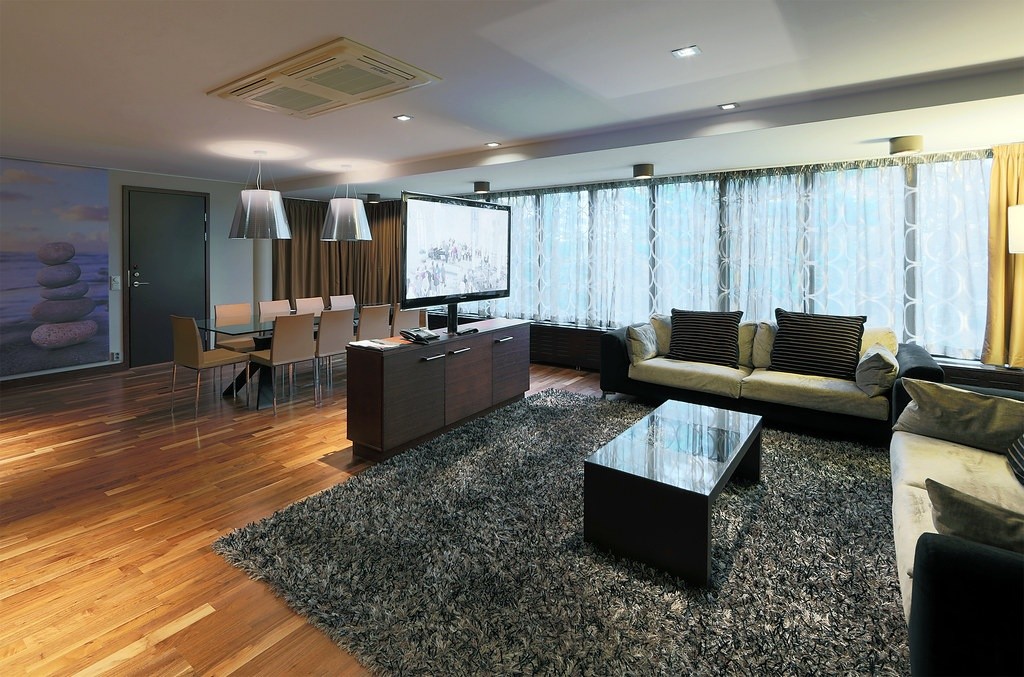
[924,476,1023,552]
[854,342,900,399]
[893,377,1022,456]
[767,307,867,381]
[624,323,659,367]
[667,307,743,369]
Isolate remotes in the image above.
[455,328,478,335]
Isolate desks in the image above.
[199,310,403,408]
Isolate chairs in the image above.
[170,293,429,417]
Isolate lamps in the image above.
[321,169,373,242]
[632,163,657,177]
[229,152,291,242]
[887,133,925,153]
[472,180,490,194]
[1007,204,1024,255]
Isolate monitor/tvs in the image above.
[400,190,511,309]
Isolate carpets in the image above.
[212,379,890,677]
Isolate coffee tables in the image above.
[583,399,761,579]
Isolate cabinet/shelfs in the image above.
[345,318,532,464]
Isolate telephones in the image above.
[399,326,440,344]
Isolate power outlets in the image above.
[112,352,121,362]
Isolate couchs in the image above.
[889,377,1023,677]
[598,305,943,444]
